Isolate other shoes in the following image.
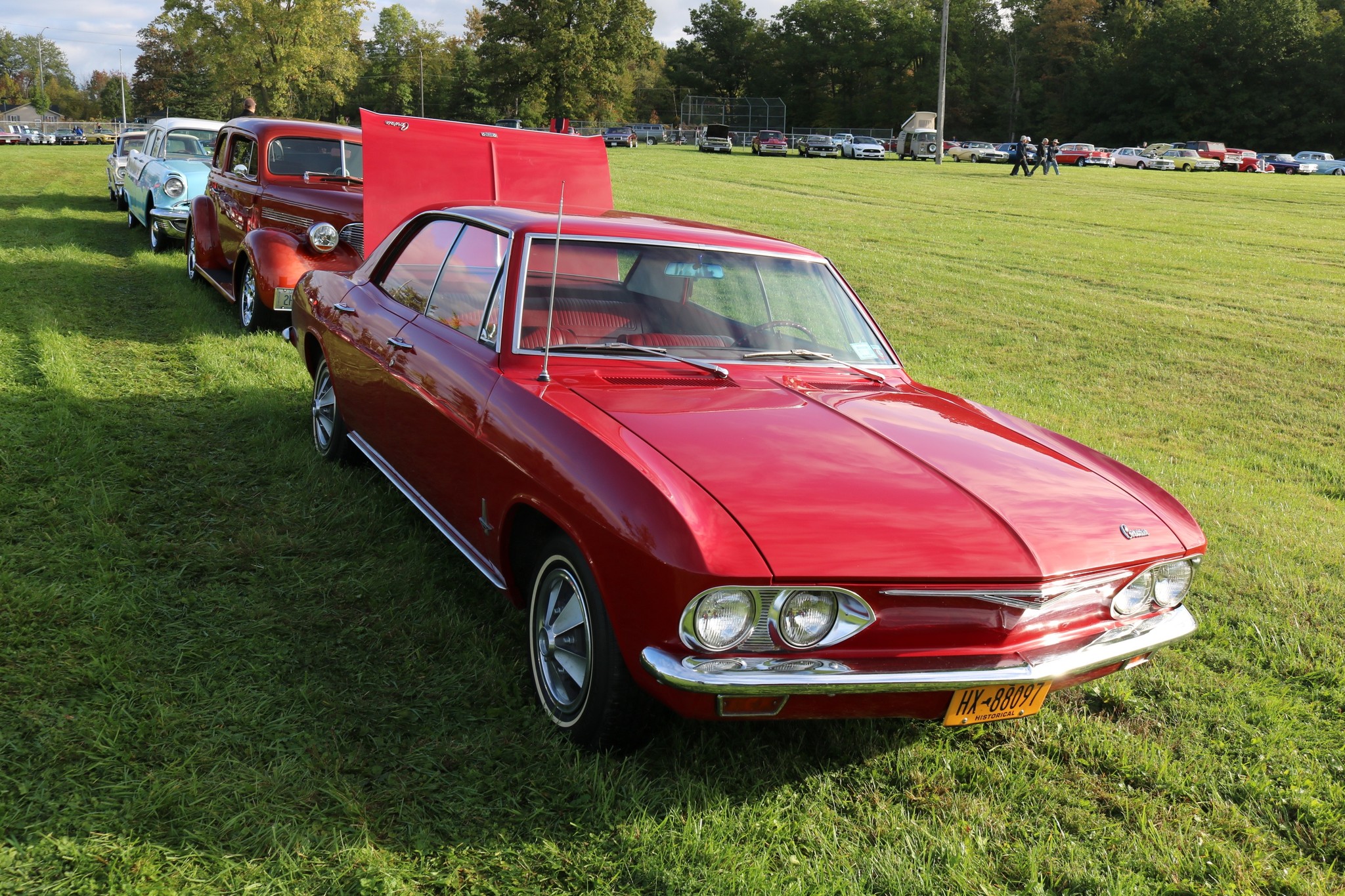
[1058,173,1061,175]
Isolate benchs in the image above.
[399,292,653,344]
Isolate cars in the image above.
[182,113,367,332]
[741,136,752,147]
[84,129,119,145]
[666,133,688,144]
[601,126,640,149]
[829,133,853,149]
[874,137,1345,177]
[566,127,581,135]
[751,130,789,156]
[840,136,885,160]
[7,125,57,146]
[116,127,170,148]
[198,137,217,151]
[280,107,1210,742]
[798,134,838,159]
[698,124,733,154]
[120,117,225,252]
[47,128,88,146]
[840,136,886,161]
[105,129,210,212]
[0,127,22,145]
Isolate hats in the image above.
[1051,139,1059,143]
[1027,136,1031,141]
[1143,142,1147,144]
[244,98,257,108]
[75,125,77,130]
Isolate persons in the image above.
[97,123,103,129]
[675,124,683,146]
[1046,139,1060,175]
[237,98,257,117]
[1009,136,1032,177]
[1137,142,1148,149]
[693,126,701,146]
[1030,138,1049,175]
[72,125,84,135]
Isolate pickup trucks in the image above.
[622,124,667,145]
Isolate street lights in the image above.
[37,25,50,131]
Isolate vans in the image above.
[895,112,945,163]
[495,119,525,130]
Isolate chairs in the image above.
[617,334,735,350]
[518,326,580,349]
